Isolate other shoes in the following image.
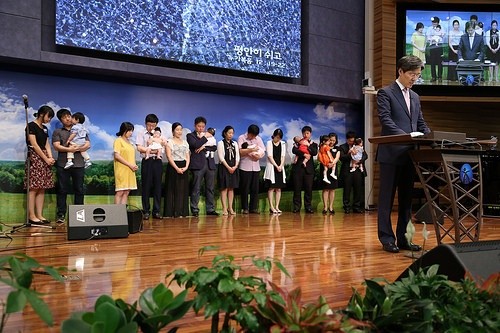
[438,78,442,83]
[55,216,65,223]
[143,213,149,220]
[322,210,327,213]
[270,208,281,213]
[416,78,425,82]
[293,209,300,212]
[345,210,350,214]
[430,77,436,82]
[249,209,260,214]
[152,213,163,219]
[353,208,362,213]
[328,208,335,214]
[29,219,43,224]
[193,212,198,217]
[306,209,313,213]
[43,219,51,222]
[207,211,219,215]
[243,210,248,214]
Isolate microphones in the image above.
[22,94,29,104]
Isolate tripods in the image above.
[4,99,56,235]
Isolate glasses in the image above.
[408,71,420,78]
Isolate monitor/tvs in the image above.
[395,1,500,96]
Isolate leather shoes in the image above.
[383,244,398,252]
[397,242,422,251]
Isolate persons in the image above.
[51,109,90,223]
[112,121,139,205]
[24,105,55,225]
[217,125,240,215]
[221,215,365,293]
[187,116,220,216]
[162,122,191,219]
[135,113,168,220]
[144,126,162,160]
[63,111,92,169]
[339,131,369,214]
[375,53,431,254]
[411,15,500,86]
[318,135,337,184]
[293,136,310,167]
[291,126,318,214]
[263,128,287,213]
[241,141,266,159]
[314,132,341,214]
[237,124,267,214]
[348,138,364,173]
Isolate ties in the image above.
[405,90,411,112]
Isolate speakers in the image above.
[389,239,500,289]
[66,205,129,239]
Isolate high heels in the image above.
[227,208,236,215]
[223,209,228,215]
